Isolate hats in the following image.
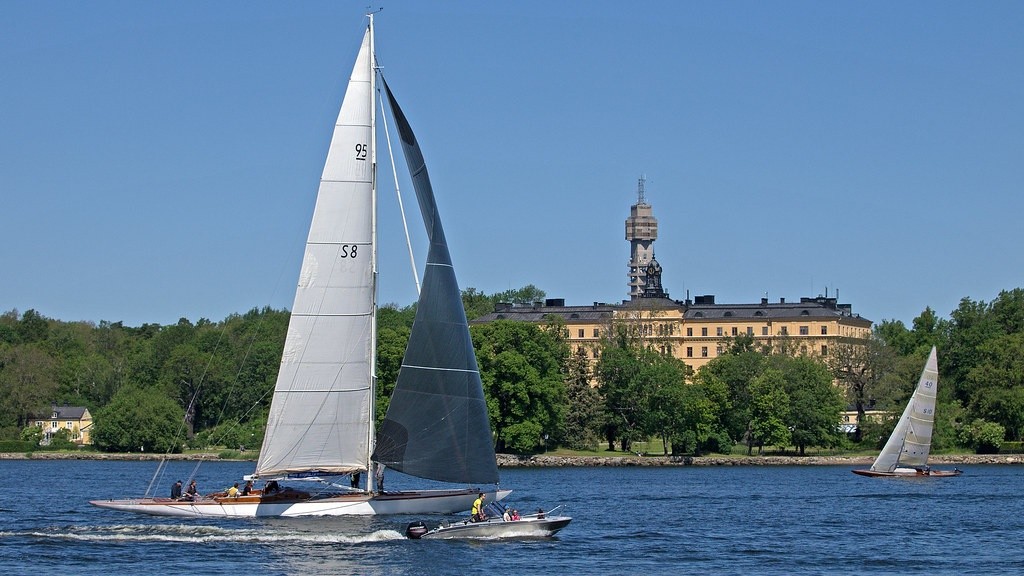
[504,504,511,510]
[178,480,183,484]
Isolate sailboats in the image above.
[852,343,963,476]
[89,14,516,524]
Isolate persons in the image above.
[184,479,201,502]
[502,505,520,521]
[472,492,487,522]
[350,461,387,494]
[922,465,930,475]
[170,480,182,502]
[228,479,278,497]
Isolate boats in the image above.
[419,504,573,543]
